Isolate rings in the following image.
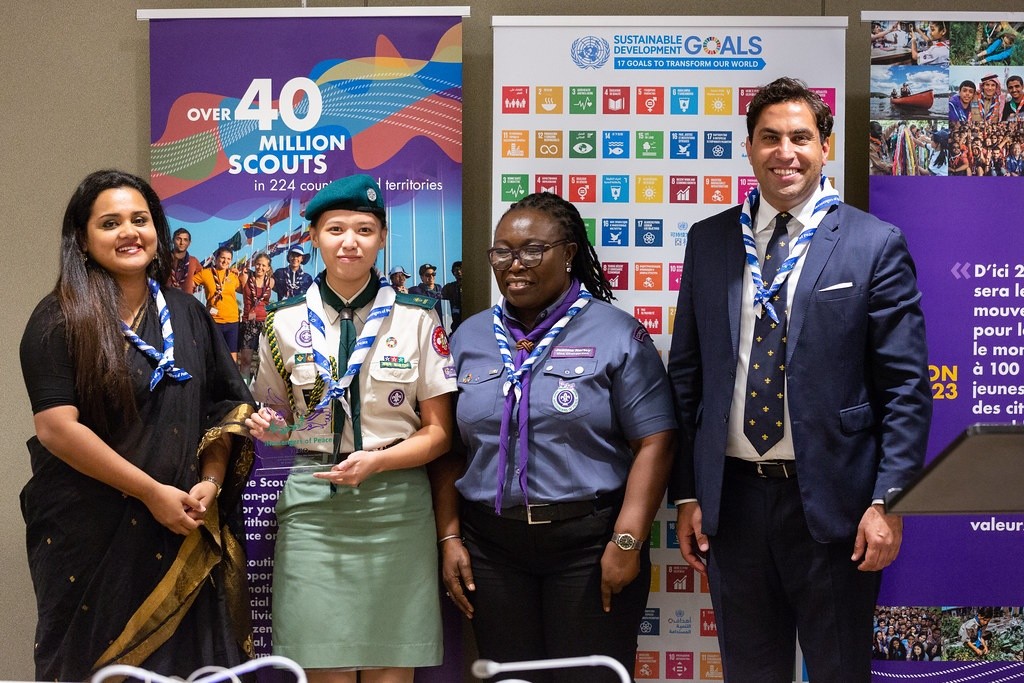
[336,478,344,482]
[446,592,450,596]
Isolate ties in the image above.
[743,211,794,455]
[495,280,580,514]
[319,272,382,499]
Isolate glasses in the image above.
[423,272,435,277]
[486,240,577,271]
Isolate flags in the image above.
[200,190,317,268]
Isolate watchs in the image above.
[202,474,222,498]
[611,532,642,551]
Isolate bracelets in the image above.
[438,535,463,542]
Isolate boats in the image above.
[890,89,935,109]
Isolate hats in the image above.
[931,129,948,142]
[451,261,462,274]
[419,264,437,275]
[304,174,384,221]
[388,266,411,280]
[286,245,310,265]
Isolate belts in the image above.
[455,486,626,525]
[299,448,351,463]
[723,454,795,478]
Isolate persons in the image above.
[890,81,912,100]
[191,245,244,369]
[270,243,314,302]
[406,264,444,327]
[970,22,1021,67]
[949,72,1024,177]
[162,228,204,296]
[240,249,276,323]
[426,193,679,683]
[438,260,465,338]
[870,21,950,66]
[665,75,932,683]
[243,171,458,683]
[871,605,941,663]
[868,119,949,177]
[388,264,411,294]
[18,167,256,683]
[957,605,993,655]
